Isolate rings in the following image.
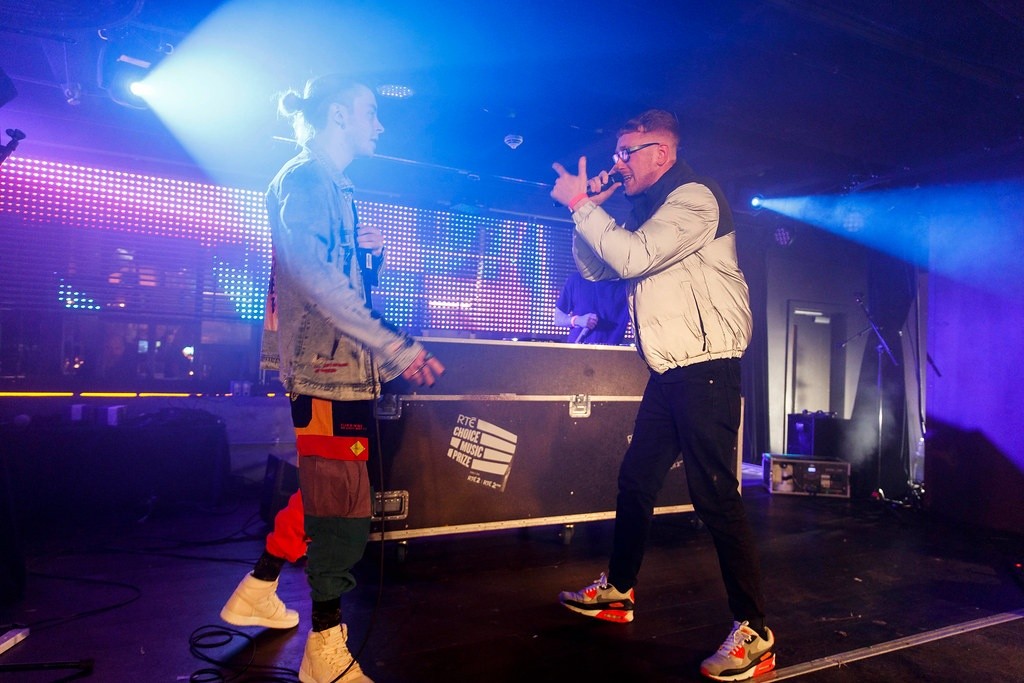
[419,363,428,371]
[423,351,434,363]
[412,369,420,376]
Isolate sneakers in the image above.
[700,620,775,681]
[558,571,635,623]
[299,623,374,683]
[219,570,299,629]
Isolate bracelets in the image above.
[571,315,579,329]
[568,193,588,213]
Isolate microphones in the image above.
[553,172,624,208]
[575,328,590,343]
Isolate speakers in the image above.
[788,414,854,460]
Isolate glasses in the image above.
[612,142,660,164]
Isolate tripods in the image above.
[852,293,917,527]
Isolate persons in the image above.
[85,334,136,389]
[550,110,774,681]
[219,74,445,683]
[554,272,629,348]
